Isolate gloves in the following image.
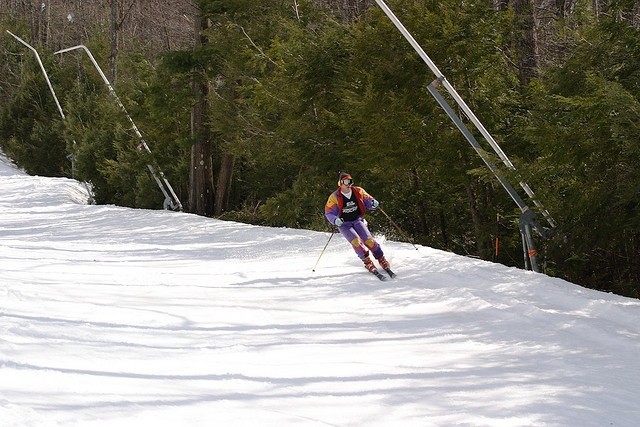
[370,199,378,207]
[334,217,343,226]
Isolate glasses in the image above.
[339,178,351,184]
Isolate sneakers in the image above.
[378,255,388,268]
[362,257,376,271]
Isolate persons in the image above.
[323,169,392,274]
